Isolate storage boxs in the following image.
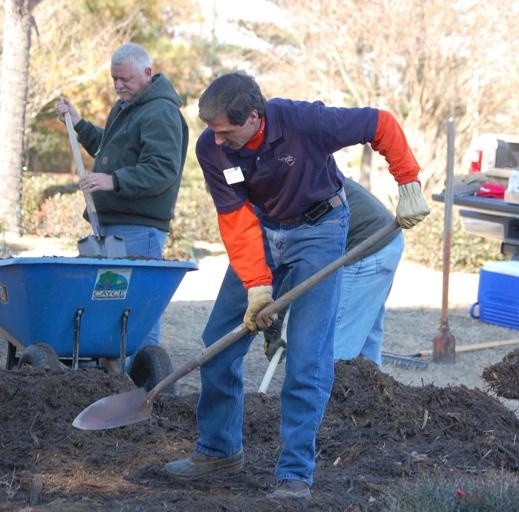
[476,258,519,330]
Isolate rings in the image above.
[92,181,96,186]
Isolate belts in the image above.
[273,187,346,225]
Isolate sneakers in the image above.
[164,447,244,482]
[270,478,311,500]
[396,181,430,229]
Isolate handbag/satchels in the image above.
[444,175,486,195]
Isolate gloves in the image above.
[244,285,278,333]
[264,338,287,364]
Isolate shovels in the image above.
[71,219,401,431]
[432,122,456,365]
[60,96,127,257]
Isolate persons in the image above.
[54,43,190,377]
[261,176,406,373]
[161,70,432,500]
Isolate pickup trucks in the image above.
[428,132,519,261]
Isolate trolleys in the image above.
[0,256,200,400]
